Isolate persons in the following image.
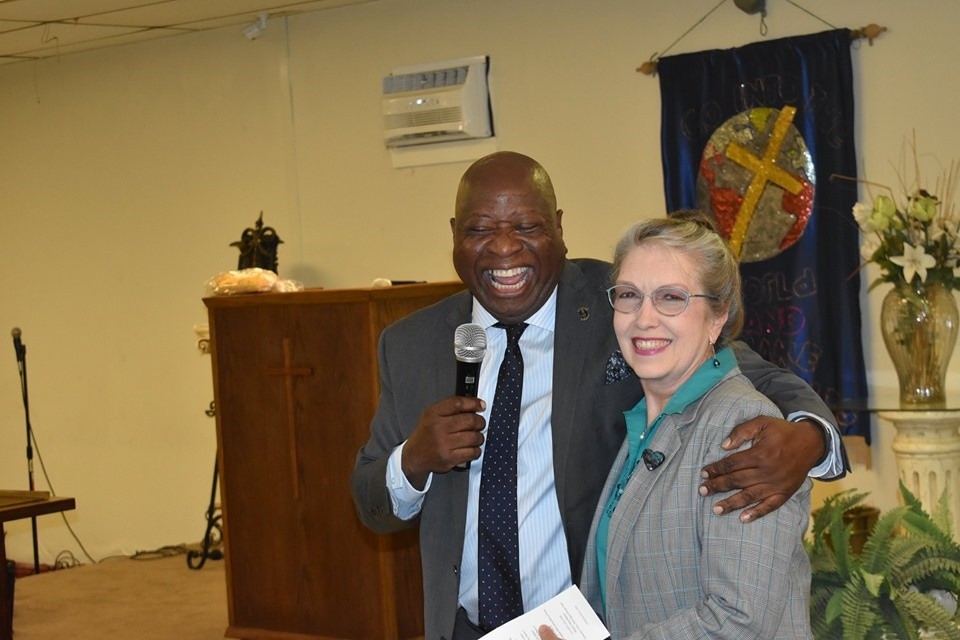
[541,207,813,640]
[351,152,852,640]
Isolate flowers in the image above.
[830,123,960,291]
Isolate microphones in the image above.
[12,327,23,376]
[454,323,487,470]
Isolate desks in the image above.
[878,410,960,543]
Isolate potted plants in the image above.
[811,502,881,559]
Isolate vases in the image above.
[880,282,959,410]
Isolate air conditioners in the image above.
[383,56,492,151]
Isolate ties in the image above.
[478,321,530,633]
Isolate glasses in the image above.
[605,284,716,316]
